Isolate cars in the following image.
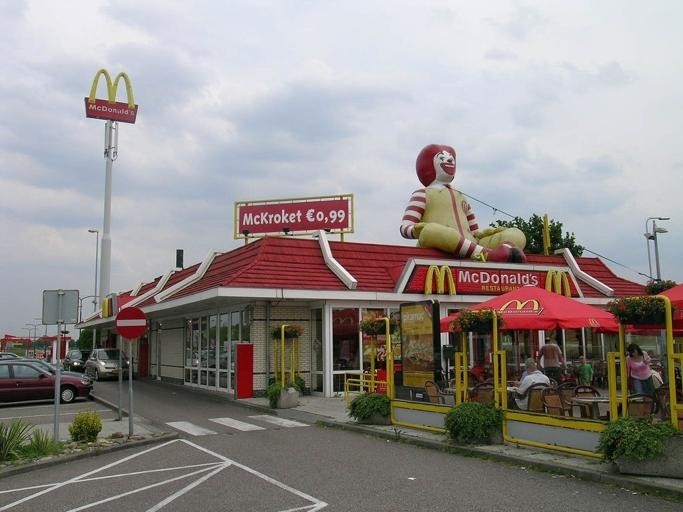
[184,344,235,381]
[0,350,95,406]
[83,346,136,383]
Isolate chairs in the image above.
[425,381,682,431]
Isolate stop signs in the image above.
[115,306,148,340]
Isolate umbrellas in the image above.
[605,283,683,335]
[438,283,620,361]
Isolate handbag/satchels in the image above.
[650,368,664,389]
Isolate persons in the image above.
[575,356,594,391]
[625,343,655,397]
[398,144,527,264]
[518,357,542,384]
[536,336,568,387]
[507,361,550,412]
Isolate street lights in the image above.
[650,220,669,283]
[76,295,100,338]
[87,228,99,351]
[20,315,49,361]
[643,216,671,286]
